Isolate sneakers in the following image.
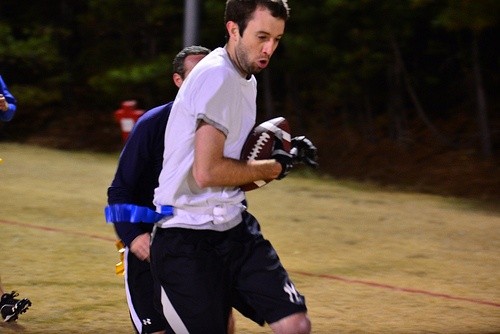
[0,290,32,324]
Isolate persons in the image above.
[107,45,235,334]
[150,0,318,334]
[0,75,31,322]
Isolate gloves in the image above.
[291,135,319,168]
[272,138,298,181]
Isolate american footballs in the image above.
[239,117,291,192]
